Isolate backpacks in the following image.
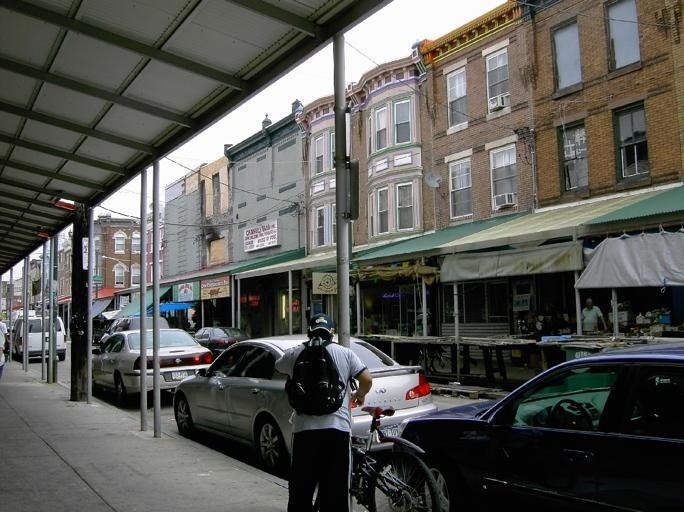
[284,340,352,416]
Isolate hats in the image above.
[307,313,334,334]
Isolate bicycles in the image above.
[350,406,441,511]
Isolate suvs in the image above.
[100,317,169,342]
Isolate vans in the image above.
[12,317,68,362]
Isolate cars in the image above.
[92,327,213,406]
[401,346,682,510]
[174,333,431,470]
[192,327,247,352]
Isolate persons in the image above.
[581,298,609,335]
[0,318,11,378]
[271,312,373,512]
